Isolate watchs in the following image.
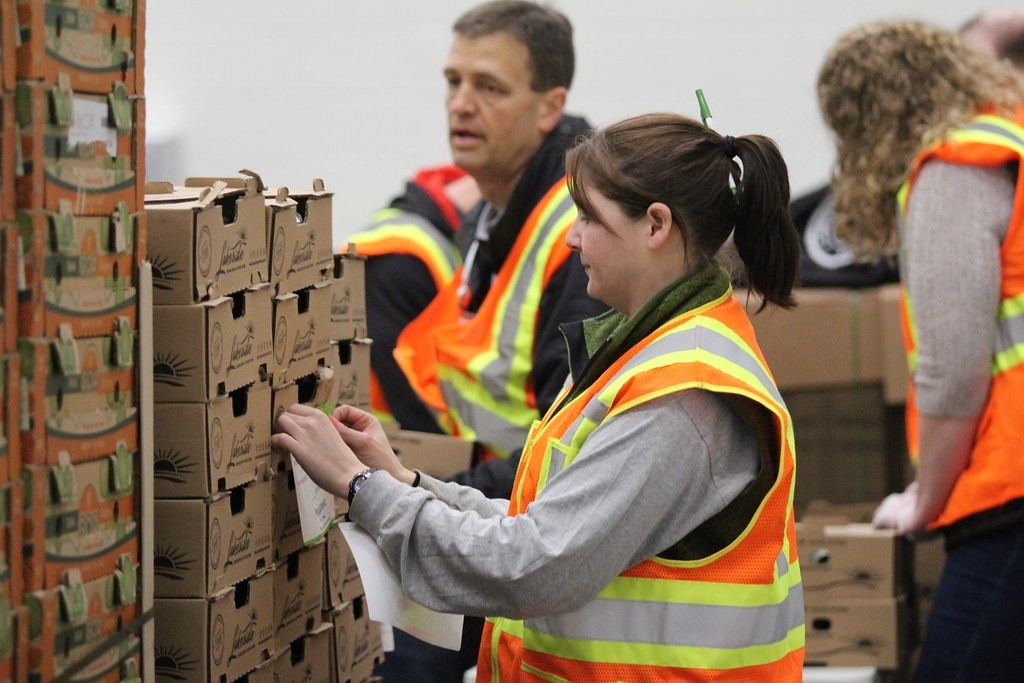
[348,469,376,506]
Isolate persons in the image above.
[337,161,484,436]
[369,0,612,683]
[270,111,805,683]
[814,18,1024,683]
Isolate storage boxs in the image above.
[1,0,387,683]
[794,500,948,670]
[732,285,907,506]
